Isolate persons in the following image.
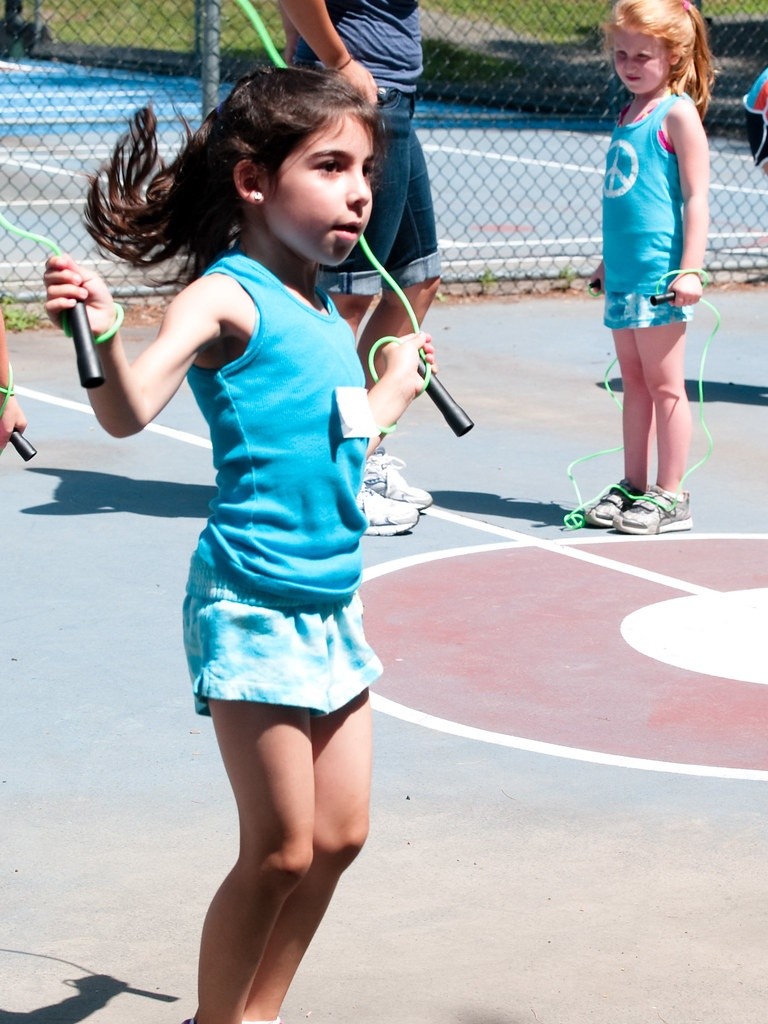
[0,309,27,453]
[43,68,437,1024]
[277,0,440,536]
[588,0,714,535]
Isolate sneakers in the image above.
[612,484,693,534]
[584,479,652,527]
[355,445,433,511]
[354,483,421,536]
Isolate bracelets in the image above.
[338,58,351,70]
